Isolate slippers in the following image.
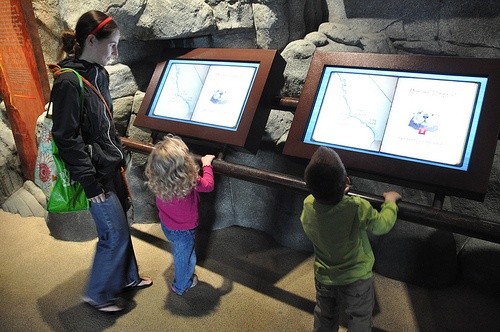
[117,276,153,291]
[83,299,126,315]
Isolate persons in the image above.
[300,147,401,332]
[52,10,153,313]
[146,134,215,295]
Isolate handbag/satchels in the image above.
[34,116,59,200]
[47,138,90,213]
[119,166,134,222]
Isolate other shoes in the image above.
[171,275,198,293]
